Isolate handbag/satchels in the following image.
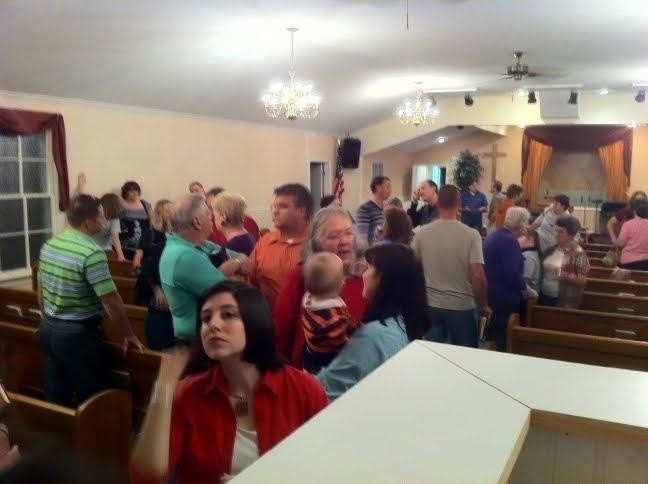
[602,239,621,267]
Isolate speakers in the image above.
[341,138,361,168]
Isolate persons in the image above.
[126,278,332,484]
[34,193,149,410]
[0,381,21,475]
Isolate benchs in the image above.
[508,232,647,373]
[0,237,163,483]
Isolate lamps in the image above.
[424,88,477,109]
[631,83,648,104]
[524,83,583,106]
[259,22,324,123]
[395,77,445,133]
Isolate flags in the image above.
[331,143,345,205]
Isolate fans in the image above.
[491,48,558,86]
